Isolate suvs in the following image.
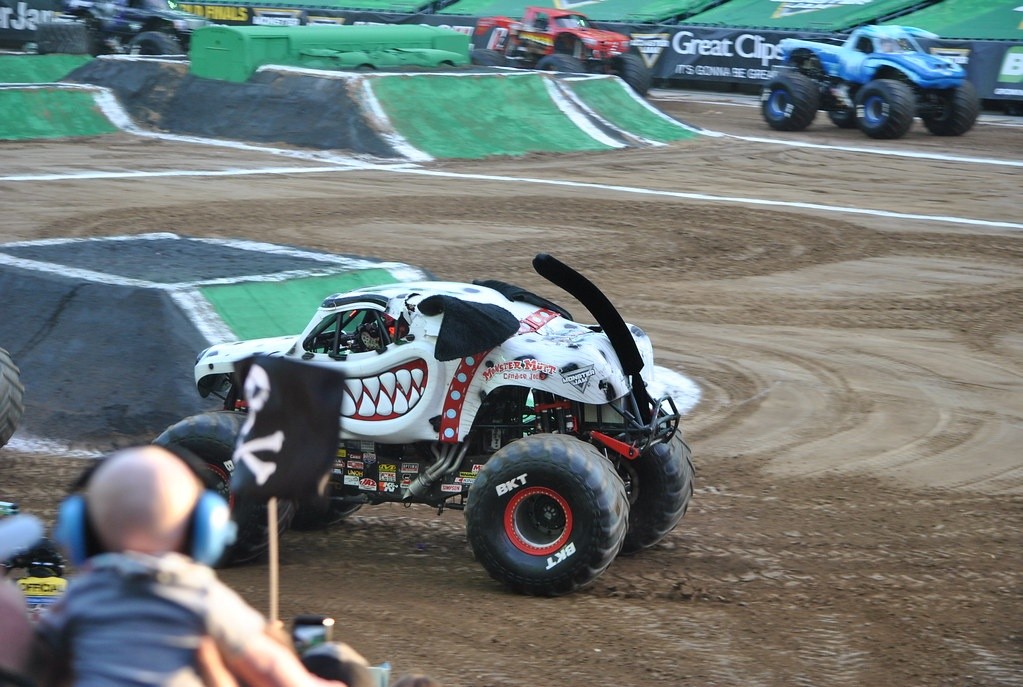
[469,5,651,97]
[760,25,981,140]
[59,0,216,56]
[144,252,696,597]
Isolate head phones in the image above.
[53,443,239,569]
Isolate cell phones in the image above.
[292,616,335,655]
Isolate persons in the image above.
[0,442,440,687]
